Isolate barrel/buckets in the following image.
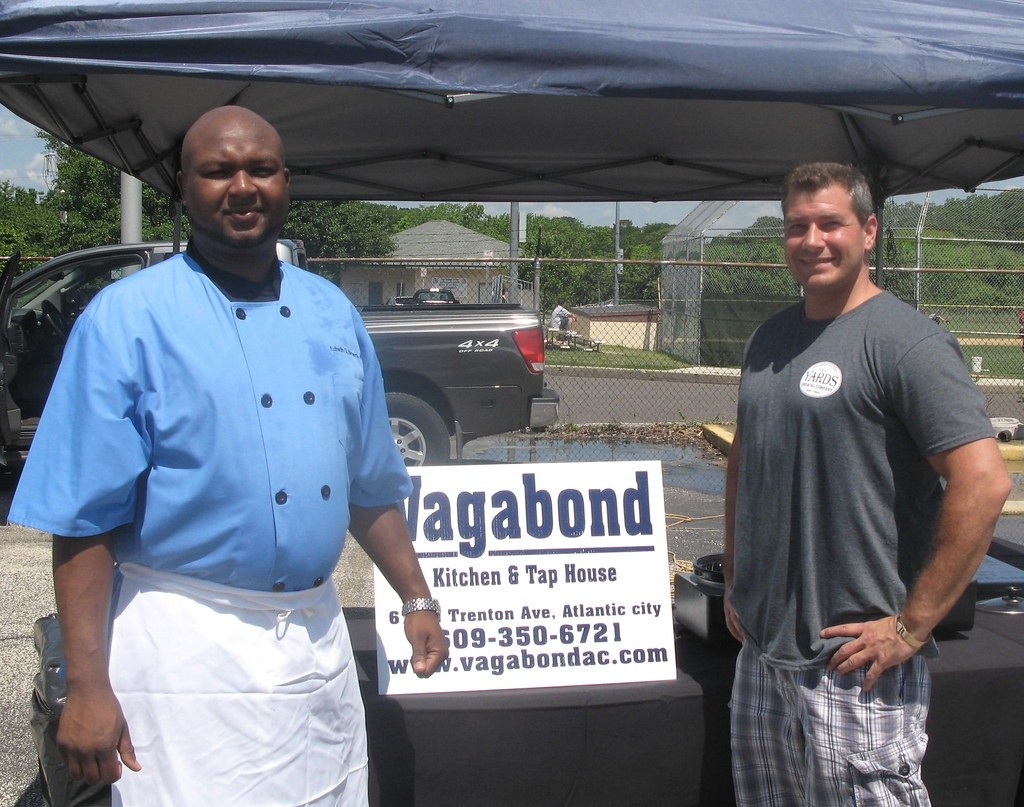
[972,357,982,372]
[990,418,1020,438]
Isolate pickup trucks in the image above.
[0,236,561,470]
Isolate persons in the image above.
[550,298,578,334]
[721,160,1014,807]
[502,287,509,303]
[6,104,448,807]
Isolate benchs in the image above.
[581,334,607,350]
[559,334,583,350]
[543,324,559,351]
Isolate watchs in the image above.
[399,597,442,624]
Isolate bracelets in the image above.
[892,613,932,648]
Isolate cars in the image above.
[384,295,413,307]
[413,287,460,305]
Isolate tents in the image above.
[0,2,1022,202]
